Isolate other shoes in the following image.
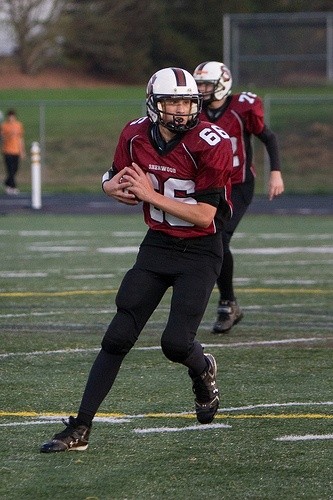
[5,186,20,195]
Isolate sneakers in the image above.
[188,353,219,424]
[211,298,244,335]
[40,416,93,453]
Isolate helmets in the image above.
[145,68,203,133]
[193,61,232,103]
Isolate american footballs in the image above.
[122,166,142,202]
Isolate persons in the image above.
[39,67,236,451]
[192,59,285,335]
[1,110,25,194]
